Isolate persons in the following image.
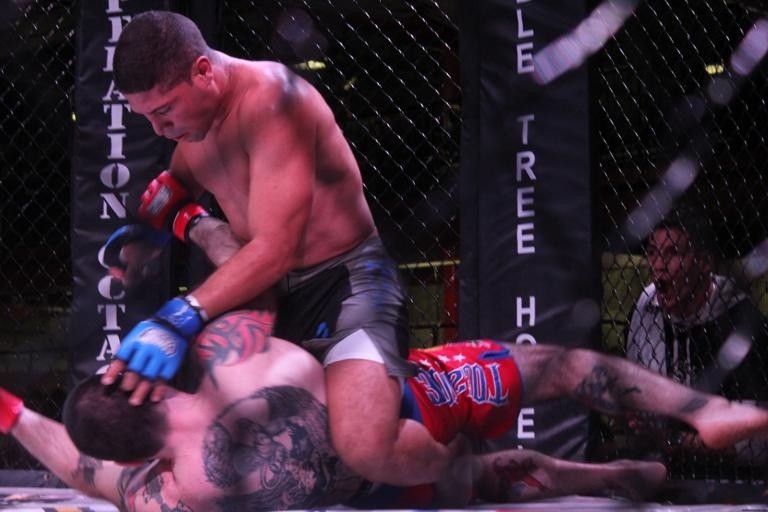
[100,10,671,512]
[626,213,752,387]
[1,172,768,512]
